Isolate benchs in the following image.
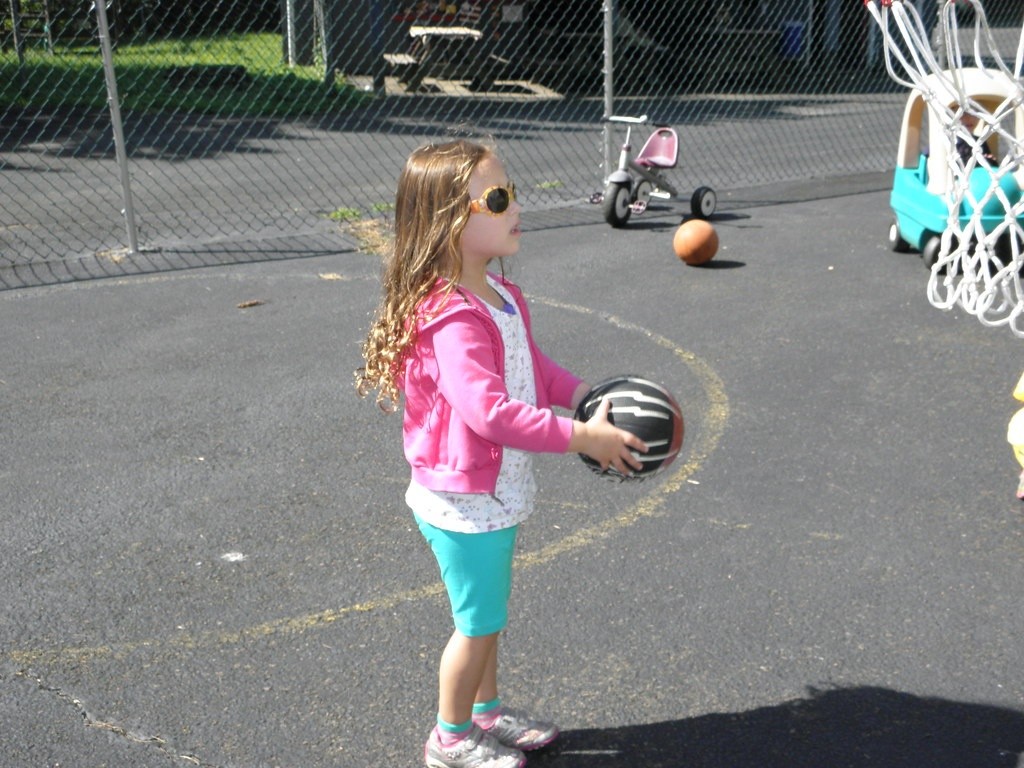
[467,54,509,91]
[383,53,428,93]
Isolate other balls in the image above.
[673,218,720,267]
[571,372,687,486]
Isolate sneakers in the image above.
[425,713,559,768]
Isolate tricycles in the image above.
[600,112,716,229]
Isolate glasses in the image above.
[470,180,516,217]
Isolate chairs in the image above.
[635,127,680,168]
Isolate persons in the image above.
[351,139,651,768]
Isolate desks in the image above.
[392,25,493,92]
